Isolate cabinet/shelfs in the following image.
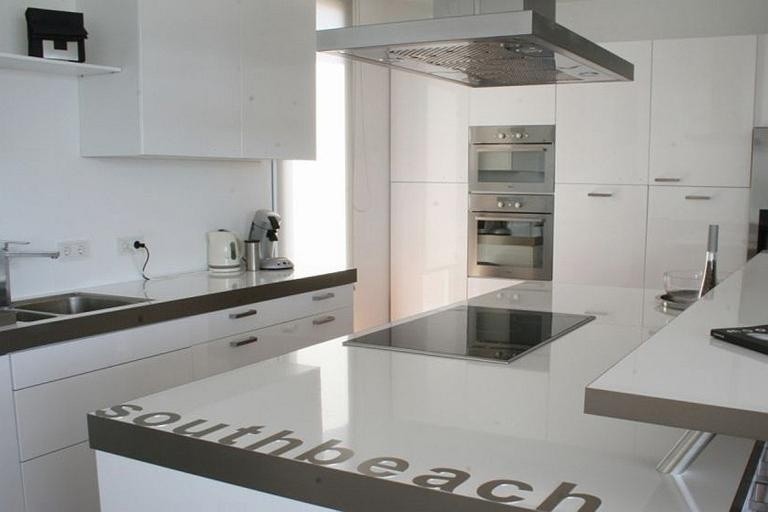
[80,0,317,162]
[0,281,354,512]
[388,33,758,324]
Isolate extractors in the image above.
[312,0,635,91]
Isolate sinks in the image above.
[0,306,56,327]
[13,291,158,313]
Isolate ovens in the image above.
[465,123,557,282]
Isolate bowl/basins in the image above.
[663,267,705,301]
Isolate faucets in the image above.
[0,239,61,306]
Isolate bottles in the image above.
[698,223,720,298]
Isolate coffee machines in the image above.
[248,209,295,271]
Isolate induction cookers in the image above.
[341,303,595,364]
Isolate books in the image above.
[709,324,767,355]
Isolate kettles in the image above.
[205,227,246,274]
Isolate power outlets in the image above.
[57,239,95,260]
[119,234,144,256]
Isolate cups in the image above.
[243,239,261,272]
